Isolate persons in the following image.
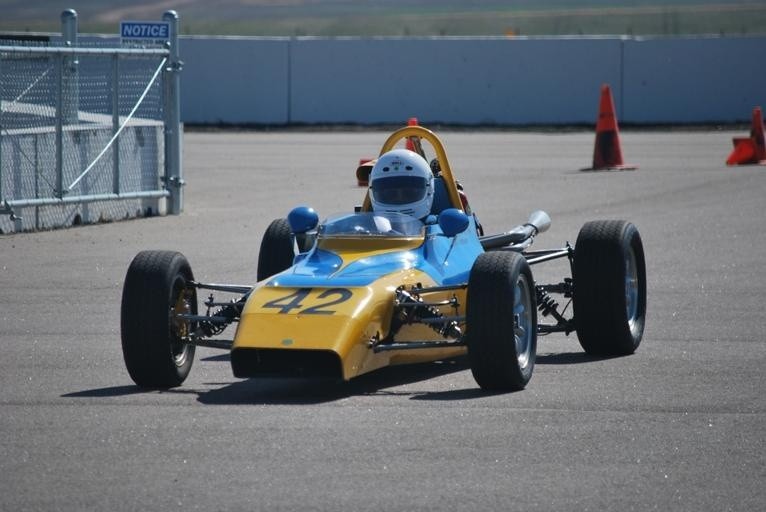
[367,147,436,237]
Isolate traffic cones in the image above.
[582,83,639,171]
[725,107,766,166]
[406,117,418,151]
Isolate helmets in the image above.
[365,147,435,220]
